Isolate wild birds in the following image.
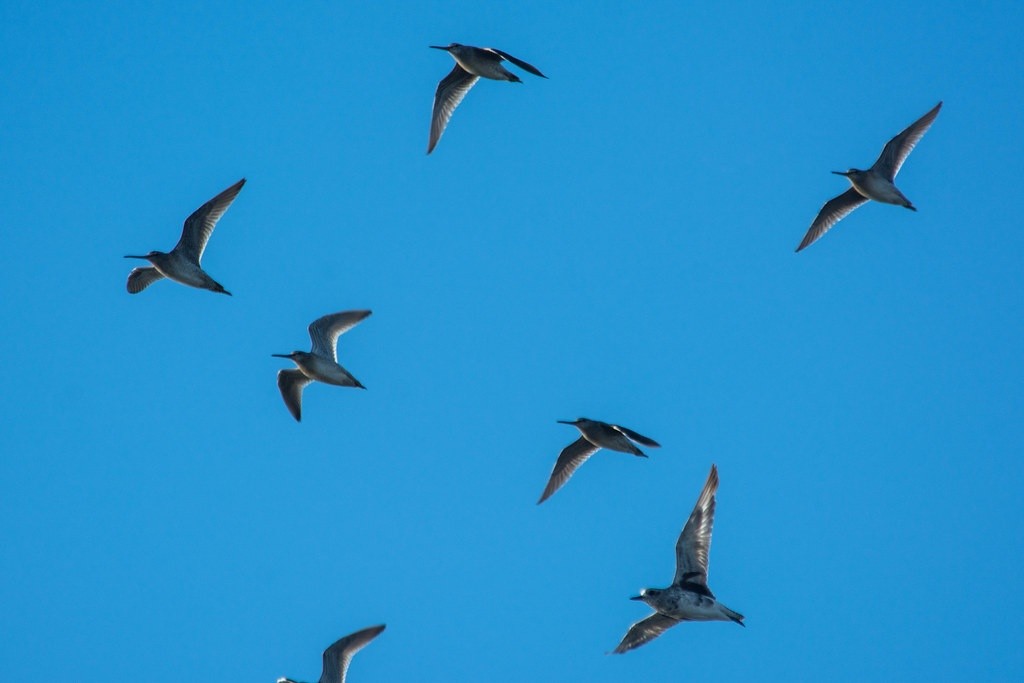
[795,101,942,253]
[426,43,548,153]
[535,418,661,506]
[124,179,247,297]
[271,310,373,423]
[276,624,387,683]
[610,464,747,654]
